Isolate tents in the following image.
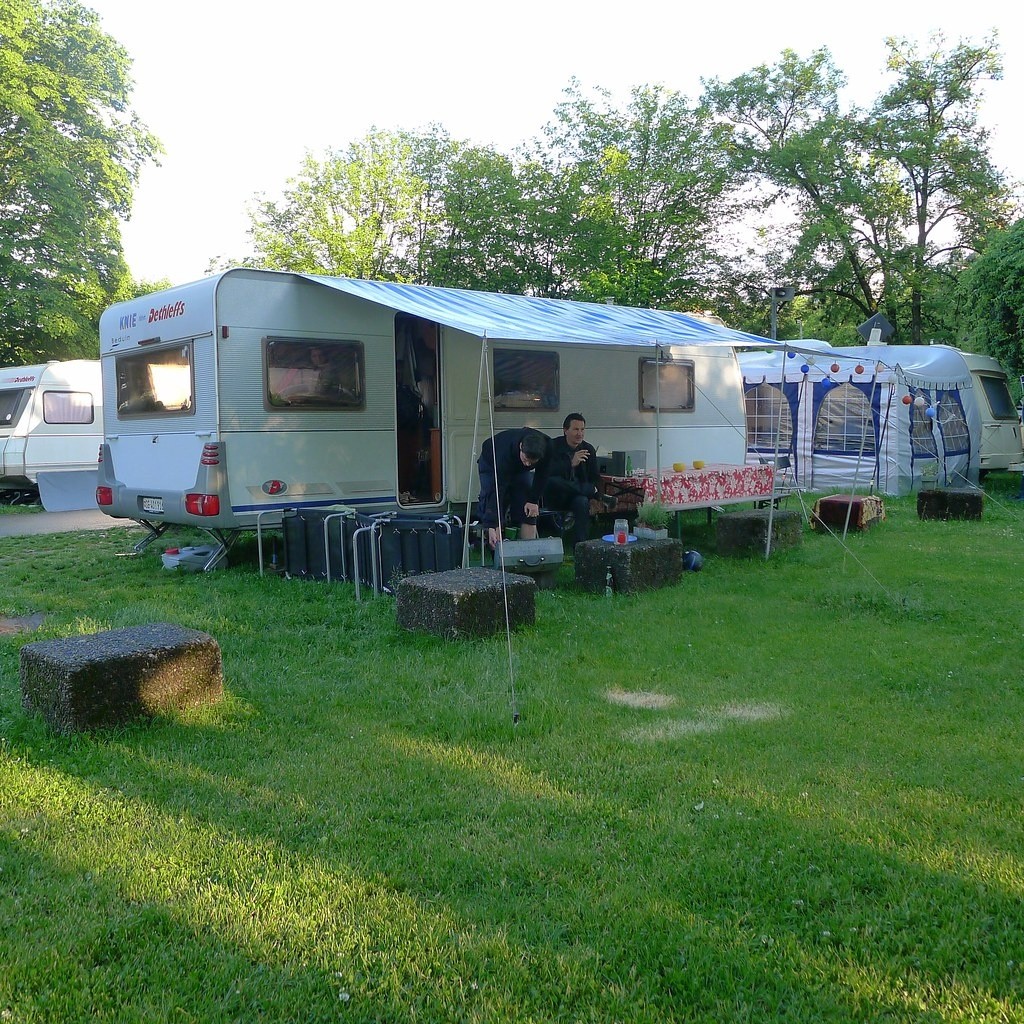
[734,341,985,499]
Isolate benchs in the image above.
[662,492,791,538]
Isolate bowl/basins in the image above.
[692,460,705,469]
[673,463,685,472]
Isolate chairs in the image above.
[759,455,809,524]
[256,472,575,603]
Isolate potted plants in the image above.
[632,499,673,541]
[920,462,939,491]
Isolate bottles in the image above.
[626,455,634,476]
[614,519,628,545]
[606,565,615,598]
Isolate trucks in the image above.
[0,358,105,506]
[927,342,1024,484]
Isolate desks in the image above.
[588,460,778,539]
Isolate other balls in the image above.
[682,549,703,571]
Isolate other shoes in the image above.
[601,492,618,510]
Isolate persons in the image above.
[477,426,553,556]
[309,347,340,393]
[540,413,618,548]
